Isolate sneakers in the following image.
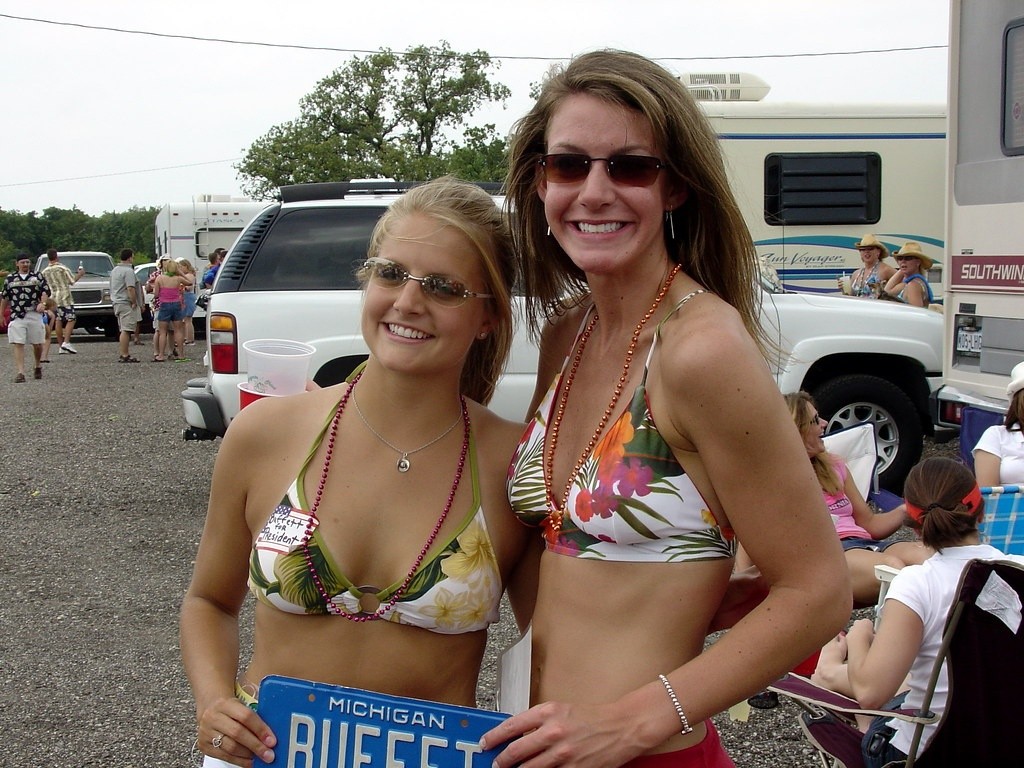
[59,342,77,354]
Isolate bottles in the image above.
[79,261,83,270]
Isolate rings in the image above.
[211,734,224,749]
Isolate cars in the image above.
[134,263,158,334]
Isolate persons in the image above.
[110,249,140,363]
[972,362,1024,487]
[811,456,1024,768]
[0,254,56,382]
[836,232,897,299]
[196,248,229,366]
[154,260,193,362]
[42,249,85,354]
[132,253,196,360]
[734,390,935,610]
[884,241,933,309]
[476,47,853,768]
[178,177,530,768]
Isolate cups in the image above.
[244,338,316,393]
[237,382,279,410]
[840,276,852,292]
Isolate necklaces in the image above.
[857,260,879,297]
[301,371,471,623]
[547,261,682,529]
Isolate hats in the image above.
[1007,361,1024,401]
[174,257,184,263]
[855,233,889,259]
[155,253,173,271]
[893,241,932,270]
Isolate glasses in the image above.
[859,248,875,252]
[798,414,820,428]
[897,256,915,261]
[364,256,497,310]
[536,152,670,188]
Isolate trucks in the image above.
[155,192,275,336]
[672,73,947,307]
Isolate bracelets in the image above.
[142,304,145,306]
[657,674,693,735]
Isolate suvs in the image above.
[34,251,121,341]
[180,181,945,498]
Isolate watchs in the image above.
[40,301,47,306]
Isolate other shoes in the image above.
[15,372,26,383]
[40,358,50,362]
[152,339,195,362]
[34,367,42,379]
[134,338,145,345]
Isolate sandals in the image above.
[119,355,140,363]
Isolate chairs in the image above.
[819,422,904,621]
[748,557,1024,768]
[978,485,1024,555]
[959,405,1007,479]
